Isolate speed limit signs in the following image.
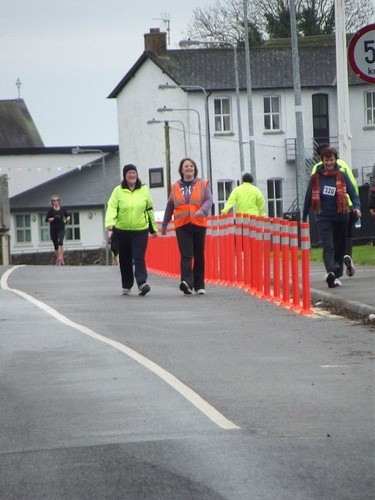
[347,22,375,84]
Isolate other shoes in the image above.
[180,281,192,295]
[334,278,342,286]
[121,288,130,295]
[343,255,355,277]
[196,289,205,295]
[55,258,64,266]
[325,272,336,288]
[138,283,150,296]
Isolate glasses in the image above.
[52,199,59,202]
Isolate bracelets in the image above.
[108,229,113,231]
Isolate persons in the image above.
[302,147,361,289]
[311,144,359,276]
[163,158,212,295]
[45,194,71,266]
[221,171,267,219]
[368,162,375,250]
[105,164,157,295]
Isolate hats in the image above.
[123,164,136,177]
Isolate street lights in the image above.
[157,106,204,179]
[146,117,187,158]
[178,37,245,185]
[72,146,110,266]
[157,81,213,193]
